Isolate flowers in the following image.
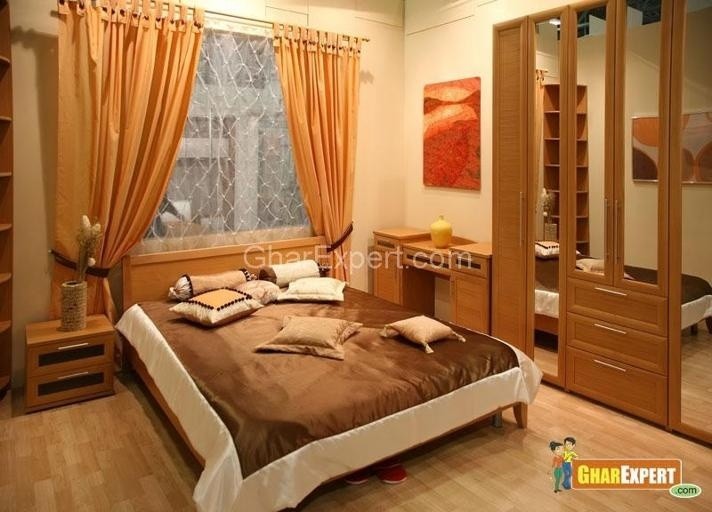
[541,186,557,216]
[73,213,102,283]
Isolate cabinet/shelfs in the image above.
[566,272,670,427]
[373,227,435,318]
[448,241,492,336]
[544,82,560,236]
[490,5,565,392]
[563,0,671,303]
[0,0,15,398]
[23,311,117,415]
[667,0,711,447]
[403,240,462,278]
[574,82,591,259]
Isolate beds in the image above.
[114,232,547,512]
[533,253,710,351]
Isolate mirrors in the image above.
[622,0,660,286]
[530,14,561,381]
[681,1,711,437]
[570,1,609,280]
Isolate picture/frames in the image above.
[629,111,712,188]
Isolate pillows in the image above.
[376,311,468,355]
[252,312,364,362]
[167,285,265,331]
[274,274,349,306]
[533,237,580,260]
[572,256,636,278]
[234,280,285,307]
[163,265,259,302]
[257,259,332,289]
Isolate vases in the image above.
[542,220,558,243]
[429,213,453,246]
[58,278,90,332]
